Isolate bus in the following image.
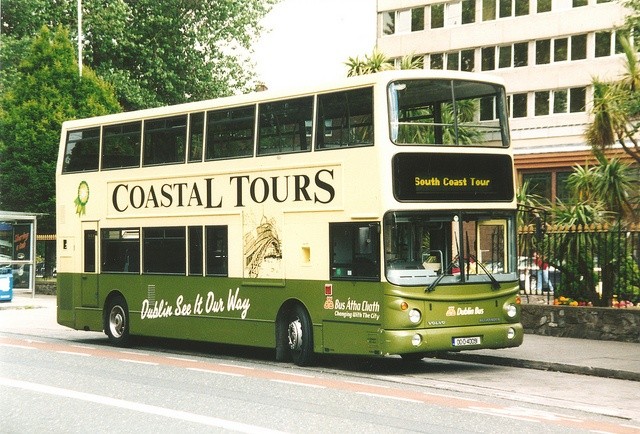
[55,69,524,366]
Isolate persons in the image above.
[533,249,554,296]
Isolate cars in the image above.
[493,256,563,296]
